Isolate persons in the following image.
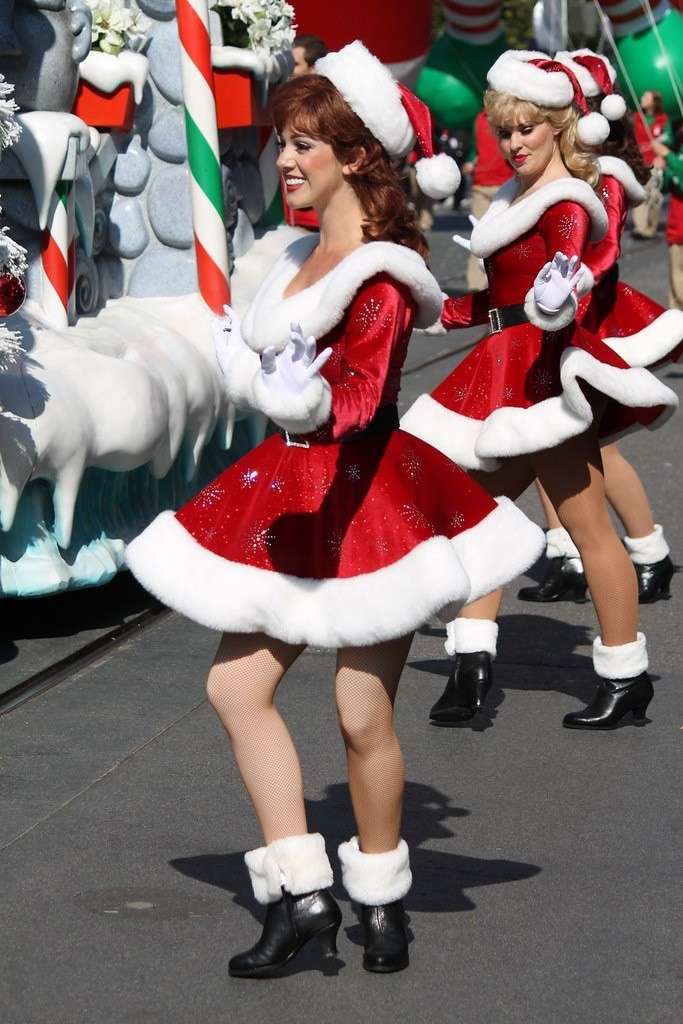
[407,147,434,233]
[518,50,682,603]
[466,105,516,293]
[400,51,678,729]
[666,101,683,312]
[282,34,329,234]
[627,90,671,239]
[124,37,546,980]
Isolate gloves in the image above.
[533,252,585,315]
[209,304,241,375]
[261,320,333,396]
[452,214,486,273]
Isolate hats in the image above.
[313,39,461,199]
[553,48,626,121]
[486,49,610,146]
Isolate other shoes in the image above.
[632,227,655,239]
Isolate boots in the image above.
[518,527,588,603]
[429,618,499,722]
[338,835,412,972]
[228,832,342,976]
[562,631,654,729]
[623,523,673,601]
